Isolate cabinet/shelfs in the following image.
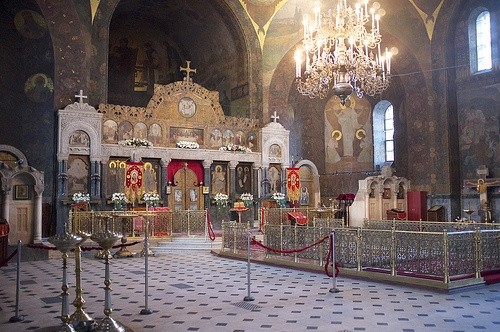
[407,190,427,220]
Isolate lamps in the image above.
[292,0,393,107]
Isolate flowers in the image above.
[72,191,91,204]
[272,192,286,205]
[219,144,252,153]
[214,192,229,208]
[240,192,254,204]
[118,137,152,147]
[112,192,128,210]
[176,140,200,149]
[140,191,160,207]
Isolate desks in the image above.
[93,212,158,259]
[230,207,249,223]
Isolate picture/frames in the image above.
[15,184,29,199]
